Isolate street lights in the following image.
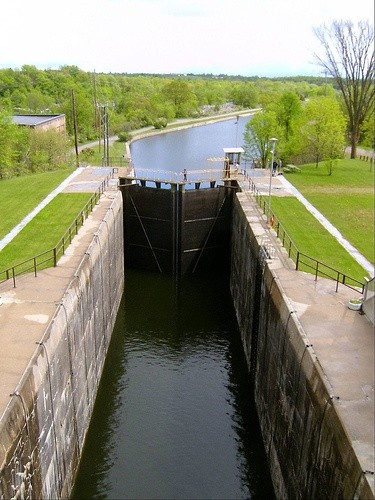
[235,115,239,167]
[267,138,278,224]
